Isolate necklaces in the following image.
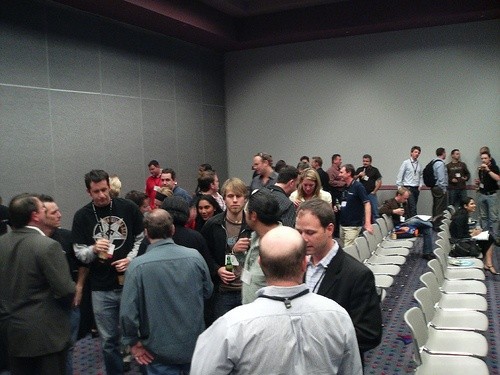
[228,216,242,223]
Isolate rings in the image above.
[136,357,139,359]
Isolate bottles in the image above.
[399,202,405,222]
[336,199,341,212]
[226,256,235,282]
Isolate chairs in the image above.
[342,205,490,375]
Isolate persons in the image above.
[379,188,443,261]
[396,146,422,216]
[422,148,449,233]
[445,149,470,206]
[0,193,87,375]
[108,151,383,328]
[474,147,500,247]
[119,209,214,375]
[70,169,145,375]
[295,200,382,375]
[449,197,500,277]
[190,226,364,375]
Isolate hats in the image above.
[258,151,273,163]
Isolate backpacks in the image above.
[390,223,419,240]
[423,159,445,188]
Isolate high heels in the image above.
[484,264,500,277]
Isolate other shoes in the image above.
[422,253,435,260]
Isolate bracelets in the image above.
[374,190,376,193]
[486,170,492,173]
